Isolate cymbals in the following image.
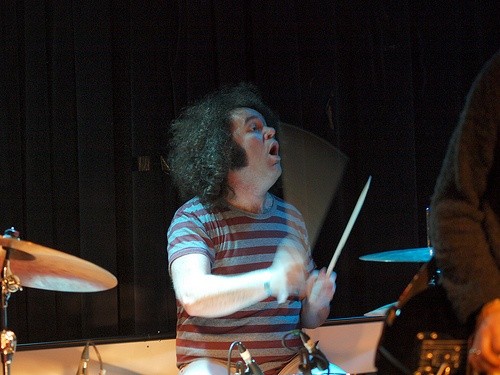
[362,302,396,315]
[0,231,120,294]
[357,246,435,263]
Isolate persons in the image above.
[426,50,500,375]
[161,85,350,375]
